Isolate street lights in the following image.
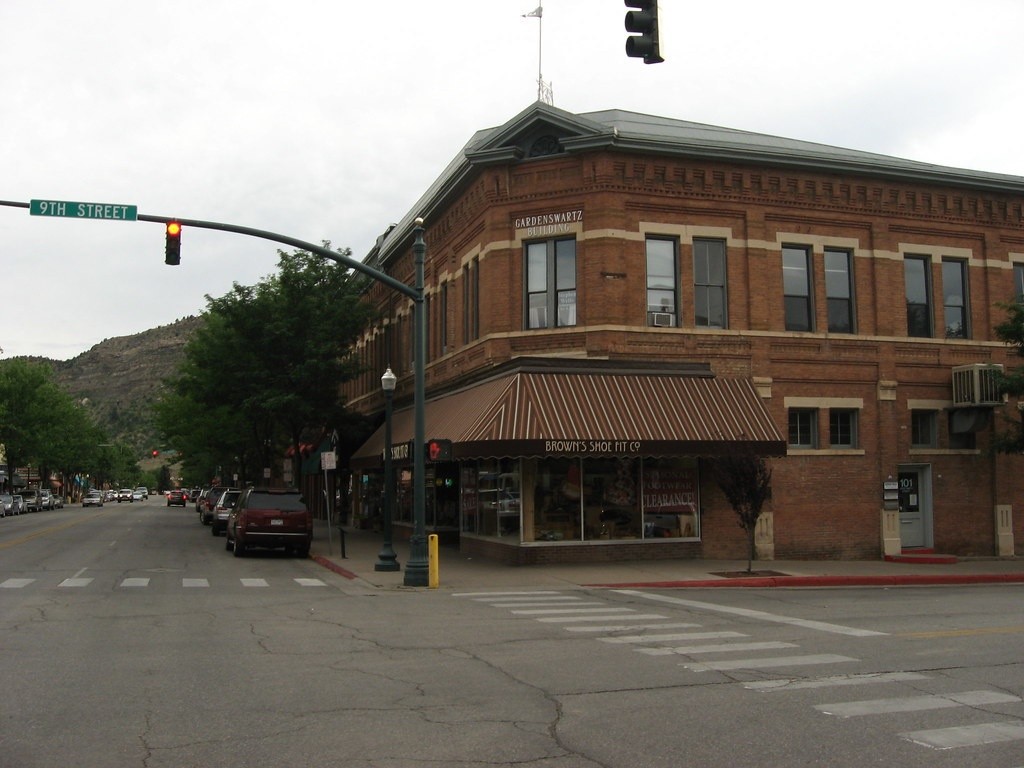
[374,363,400,571]
[27,463,31,490]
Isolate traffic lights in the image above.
[152,450,158,456]
[428,438,452,462]
[165,221,181,265]
[625,0,664,65]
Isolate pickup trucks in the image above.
[199,486,240,525]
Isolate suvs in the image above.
[118,489,134,503]
[40,488,55,511]
[135,487,148,499]
[225,486,313,559]
[211,488,242,536]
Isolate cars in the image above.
[53,494,64,509]
[89,489,118,503]
[13,495,28,515]
[82,493,103,507]
[0,495,19,518]
[133,492,143,501]
[164,488,213,513]
[16,489,42,513]
